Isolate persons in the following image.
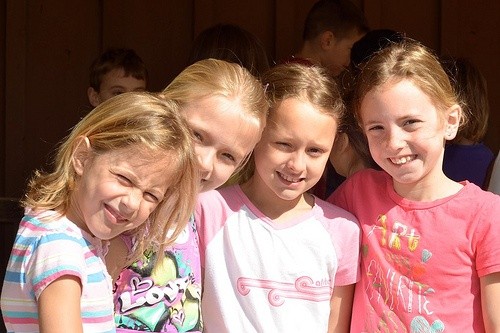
[187,22,266,75]
[435,48,495,190]
[197,63,361,332]
[328,35,499,333]
[86,47,146,108]
[281,0,407,201]
[102,58,270,332]
[0,90,200,332]
